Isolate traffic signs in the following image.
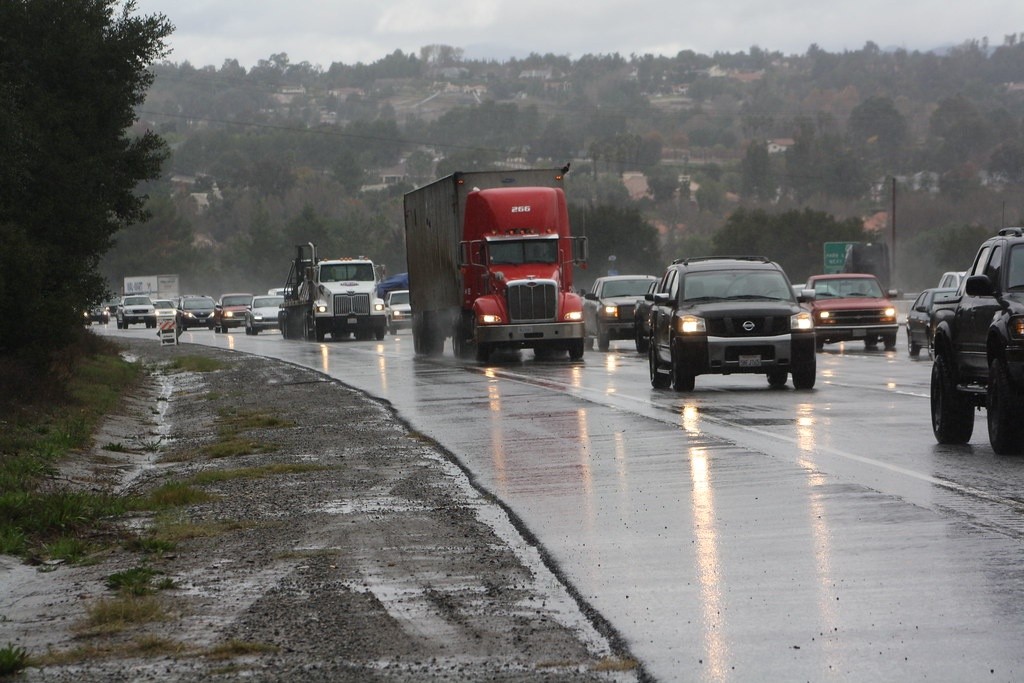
[822,241,861,274]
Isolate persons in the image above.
[534,245,545,261]
[352,266,369,282]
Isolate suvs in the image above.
[639,253,818,391]
[930,226,1024,456]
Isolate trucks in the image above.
[385,288,413,336]
[268,287,293,300]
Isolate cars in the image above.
[800,274,899,350]
[907,286,965,357]
[588,274,663,352]
[180,295,217,330]
[244,295,284,336]
[153,299,177,327]
[213,293,256,334]
[116,295,157,329]
[83,302,109,326]
[939,270,968,288]
[791,282,844,303]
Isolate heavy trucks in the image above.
[403,163,587,365]
[276,241,387,343]
